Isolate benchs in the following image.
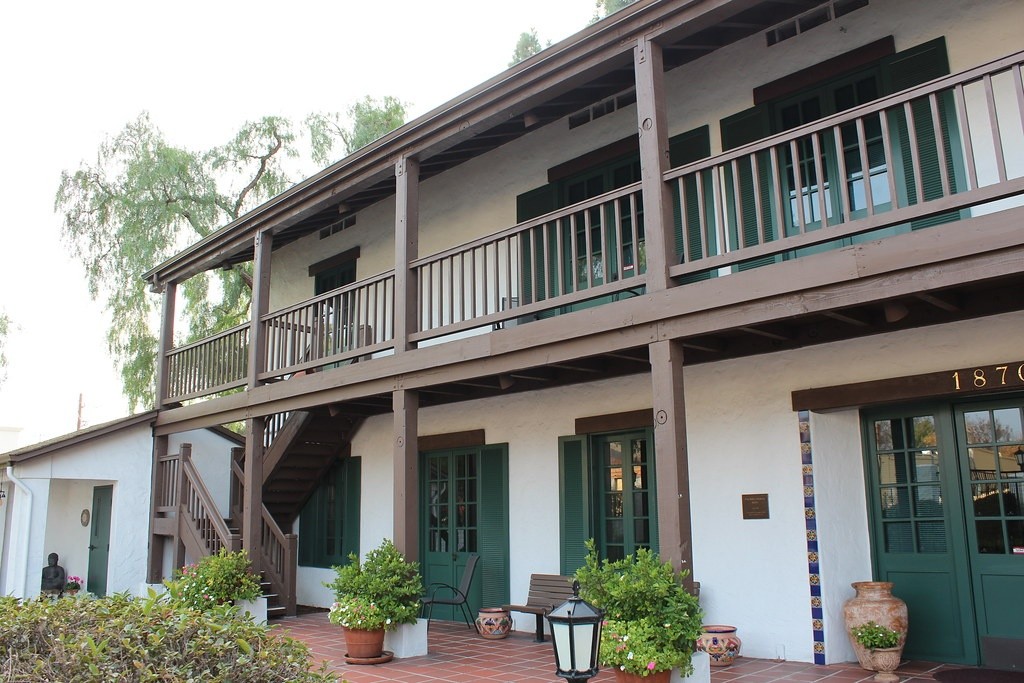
[503,571,707,641]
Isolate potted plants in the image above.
[850,621,903,683]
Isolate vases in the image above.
[699,623,741,667]
[613,666,672,683]
[340,625,387,658]
[476,606,514,640]
[846,578,909,671]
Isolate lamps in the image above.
[544,578,604,682]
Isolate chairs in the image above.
[422,553,481,633]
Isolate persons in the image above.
[41,553,65,591]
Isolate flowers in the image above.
[175,561,216,609]
[67,575,86,590]
[597,618,694,677]
[327,598,393,631]
